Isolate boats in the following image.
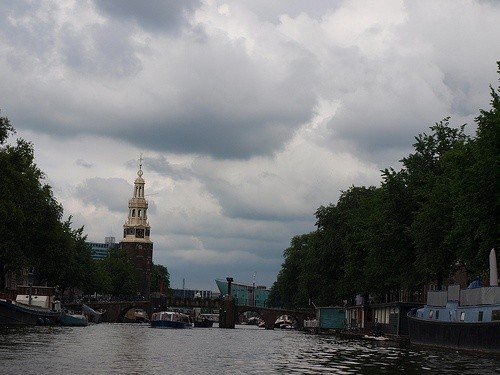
[150,312,192,327]
[0,294,89,326]
[61,310,88,325]
